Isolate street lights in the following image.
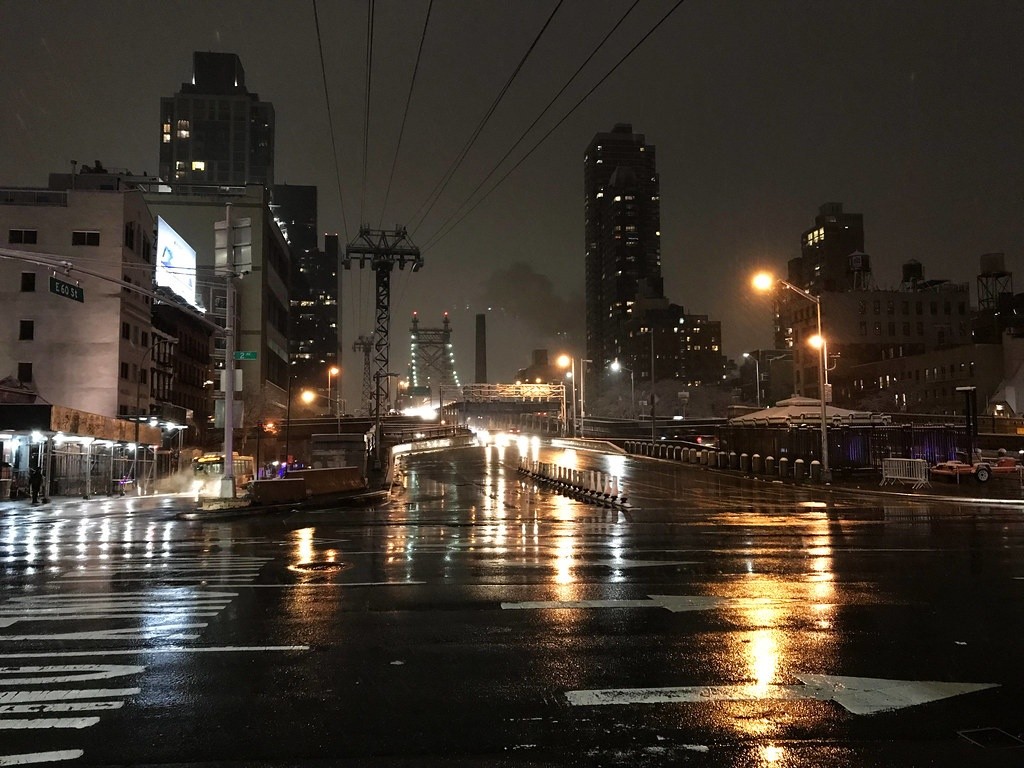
[135,338,179,444]
[256,422,274,480]
[743,353,760,408]
[641,328,656,444]
[374,372,400,470]
[263,460,278,479]
[580,358,593,436]
[302,391,341,434]
[610,363,635,418]
[555,355,575,439]
[750,271,833,483]
[328,368,338,417]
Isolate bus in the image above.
[192,451,255,491]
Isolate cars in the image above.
[930,457,1024,481]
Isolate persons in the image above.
[30,467,43,504]
